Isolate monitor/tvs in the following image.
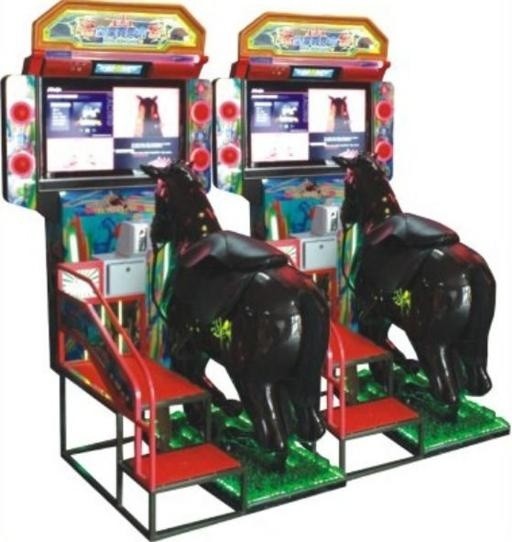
[246,83,372,174]
[43,83,182,179]
